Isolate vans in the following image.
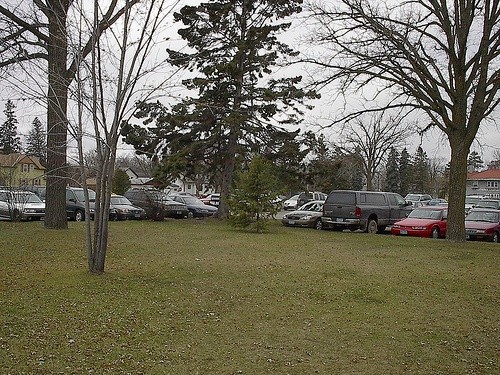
[320,189,415,234]
[0,190,45,221]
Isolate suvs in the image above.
[297,191,326,208]
[404,194,433,208]
[39,186,116,222]
[124,186,189,221]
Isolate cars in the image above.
[199,194,235,206]
[436,203,474,218]
[282,200,330,230]
[464,207,500,243]
[283,194,298,210]
[391,206,467,240]
[169,194,218,220]
[109,194,147,221]
[426,198,448,206]
[472,199,500,210]
